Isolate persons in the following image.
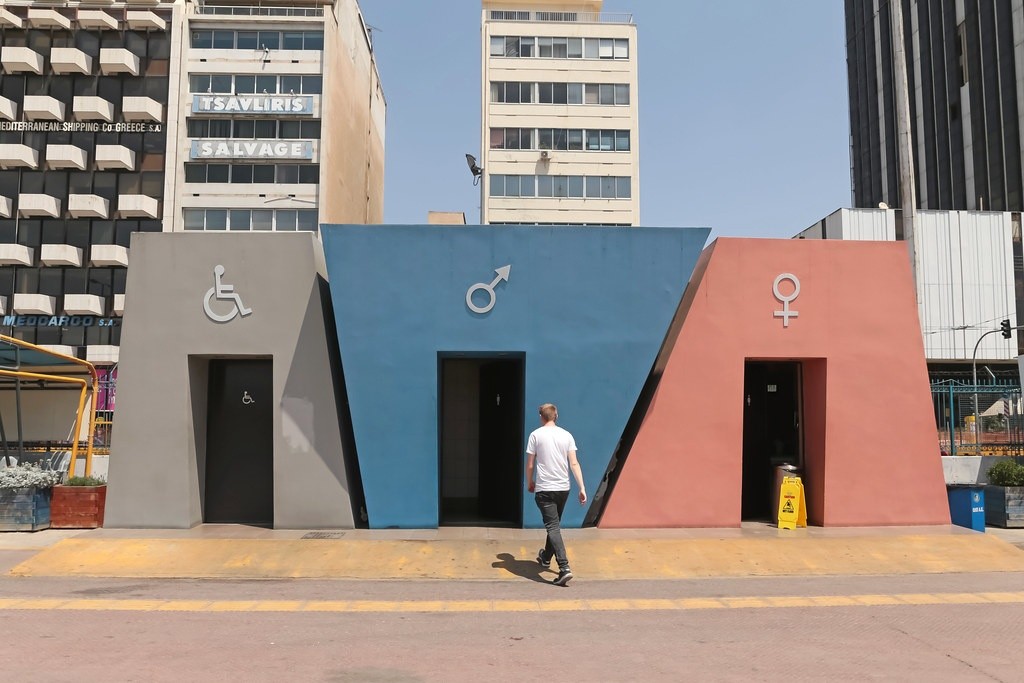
[524,404,588,586]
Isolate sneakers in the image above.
[554,568,573,585]
[539,549,551,566]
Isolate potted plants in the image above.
[50,472,106,528]
[0,458,66,531]
[978,456,1024,527]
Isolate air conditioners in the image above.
[540,150,552,160]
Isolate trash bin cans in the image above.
[947,484,986,533]
[772,462,803,524]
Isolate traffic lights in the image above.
[1001,319,1012,340]
[969,396,975,410]
